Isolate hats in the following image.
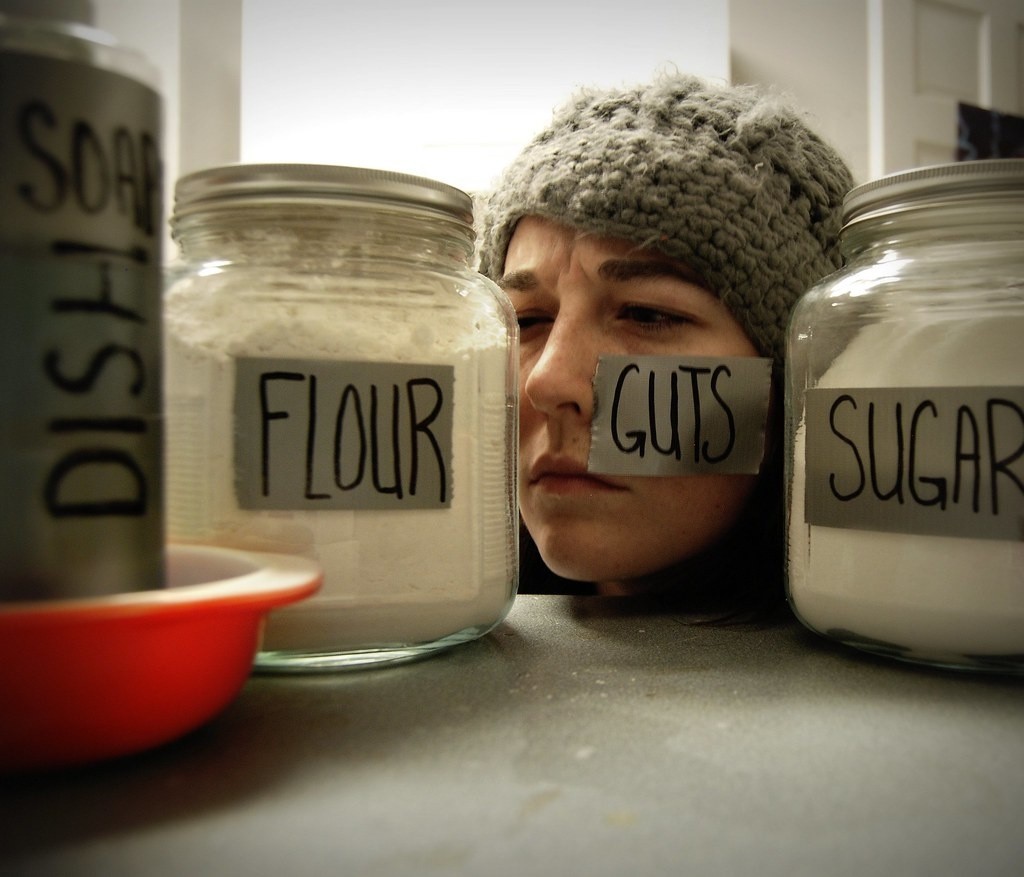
[475,70,857,401]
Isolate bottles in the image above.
[167,165,518,675]
[0,0,166,601]
[784,159,1023,676]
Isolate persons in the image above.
[478,62,860,630]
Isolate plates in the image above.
[0,544,323,760]
